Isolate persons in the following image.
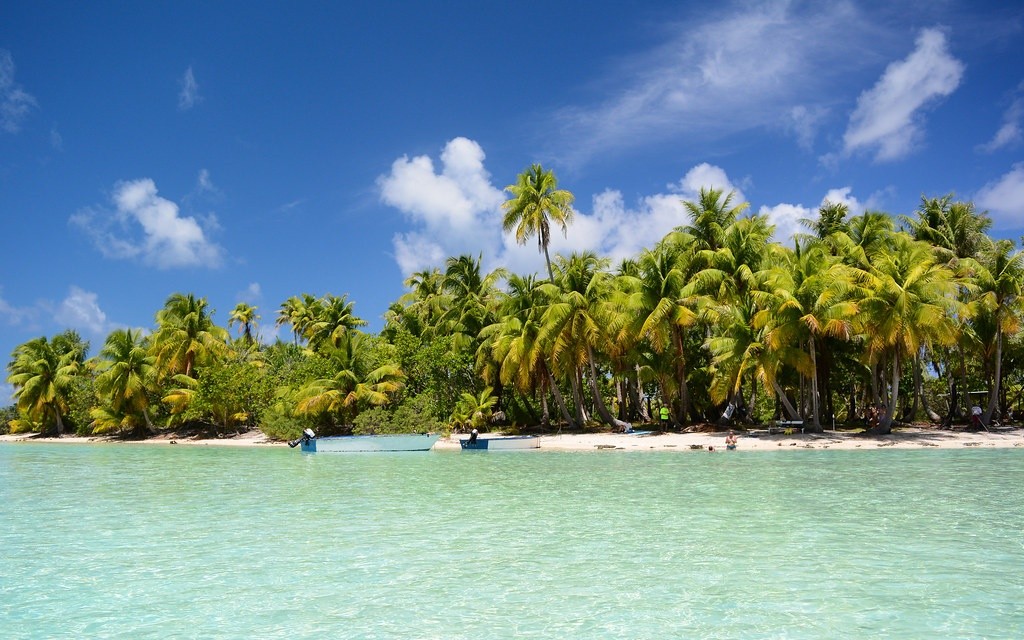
[660,403,669,431]
[709,446,715,452]
[863,403,886,427]
[972,402,982,429]
[726,430,737,449]
[1005,411,1016,420]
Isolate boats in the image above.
[459,434,542,450]
[288,428,443,452]
